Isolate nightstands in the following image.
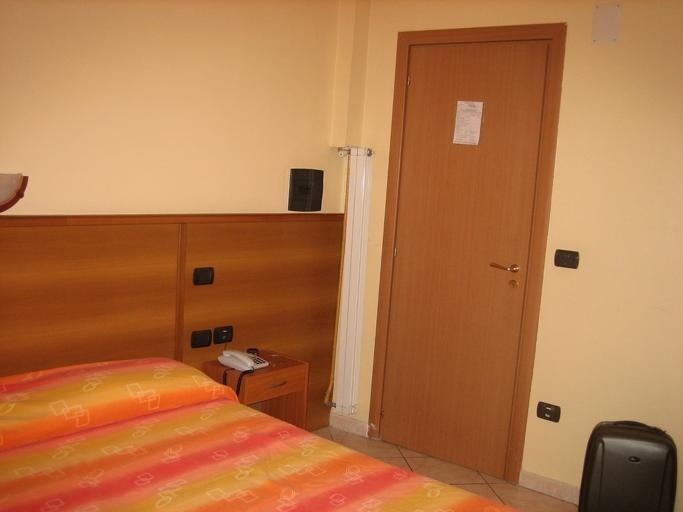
[201,350,309,430]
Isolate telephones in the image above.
[218,350,269,372]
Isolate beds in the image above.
[0,356,525,512]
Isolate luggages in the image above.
[578,419,677,512]
